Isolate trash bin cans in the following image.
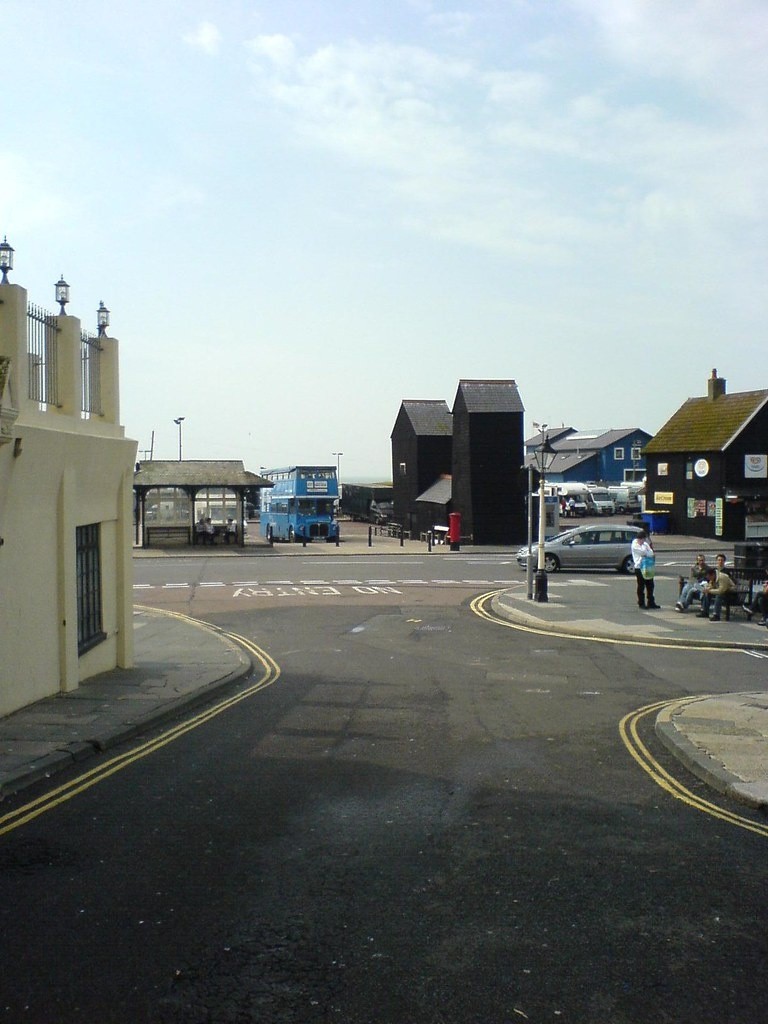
[642,510,670,535]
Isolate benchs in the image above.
[195,525,238,544]
[146,524,190,544]
[678,577,752,622]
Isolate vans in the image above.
[585,488,616,517]
[606,486,642,515]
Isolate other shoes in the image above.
[758,621,768,626]
[710,615,720,621]
[743,603,754,613]
[696,613,709,617]
[646,603,660,609]
[677,602,684,611]
[675,606,682,612]
[640,604,648,609]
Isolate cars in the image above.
[514,525,654,575]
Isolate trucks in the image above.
[537,482,589,518]
[341,483,393,525]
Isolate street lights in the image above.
[173,416,185,461]
[333,453,343,489]
[533,434,559,603]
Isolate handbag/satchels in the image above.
[640,555,655,579]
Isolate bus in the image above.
[259,465,341,543]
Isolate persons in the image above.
[195,517,236,546]
[561,496,575,516]
[631,532,661,609]
[674,554,768,629]
